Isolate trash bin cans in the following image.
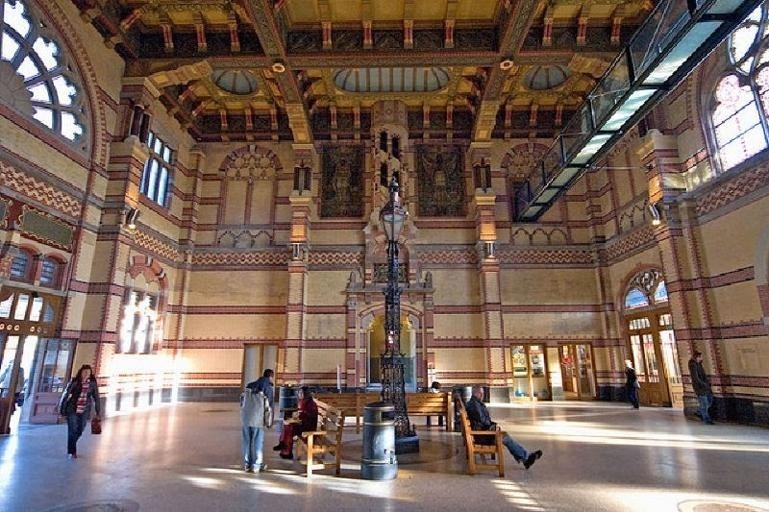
[360,401,398,480]
[276,384,300,433]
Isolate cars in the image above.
[17,377,62,406]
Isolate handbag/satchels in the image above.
[54,393,75,416]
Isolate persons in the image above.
[271,386,319,458]
[428,381,442,394]
[623,359,640,409]
[465,384,542,470]
[238,381,274,473]
[421,153,458,211]
[0,355,26,436]
[331,156,354,208]
[58,364,102,460]
[688,349,716,424]
[250,368,275,407]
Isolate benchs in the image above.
[285,398,344,474]
[313,389,452,433]
[458,396,504,474]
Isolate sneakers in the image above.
[67,452,79,459]
[523,450,543,470]
[244,464,268,474]
[272,441,294,460]
